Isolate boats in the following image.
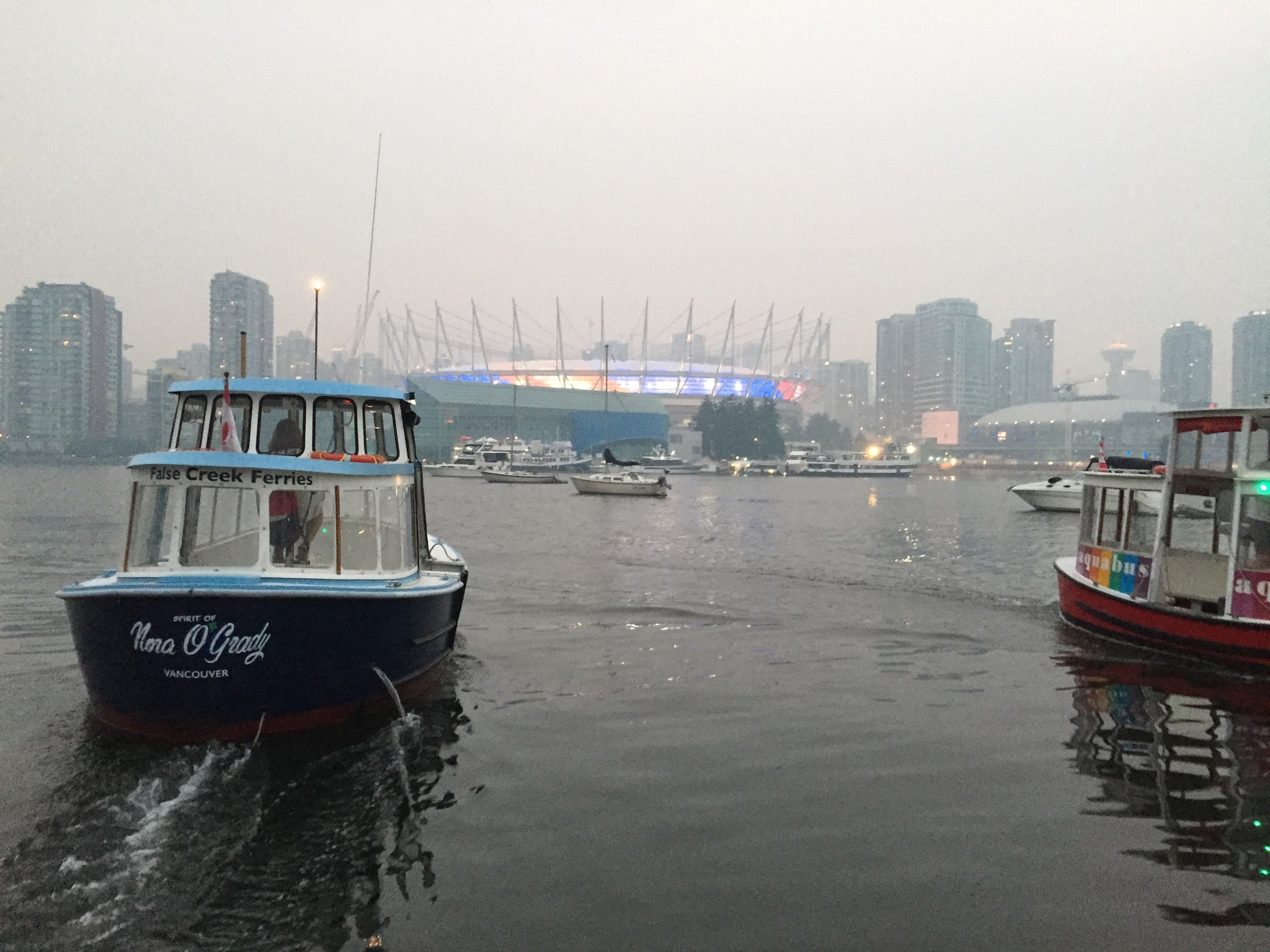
[716,440,918,478]
[589,444,703,474]
[55,279,467,746]
[569,468,673,497]
[1010,388,1270,674]
[421,388,592,484]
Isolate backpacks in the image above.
[268,489,300,547]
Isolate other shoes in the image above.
[296,542,311,560]
[273,552,285,563]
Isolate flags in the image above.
[1099,440,1107,473]
[221,379,243,452]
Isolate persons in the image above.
[1215,479,1270,569]
[1128,500,1145,545]
[269,418,325,565]
[1216,597,1225,617]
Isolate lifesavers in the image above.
[310,450,386,465]
[1153,463,1195,473]
